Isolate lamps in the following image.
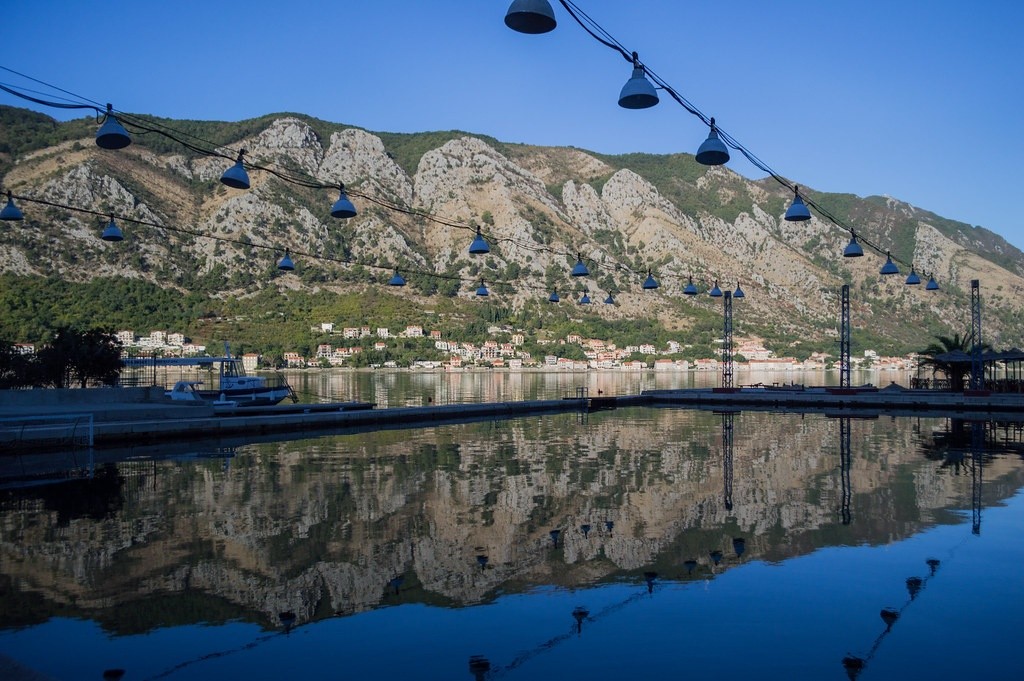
[0,199,24,222]
[330,194,357,219]
[905,270,921,284]
[469,235,491,254]
[683,283,697,296]
[548,260,614,304]
[101,220,123,242]
[95,111,132,149]
[220,159,251,190]
[880,258,899,275]
[618,65,659,110]
[710,287,723,297]
[504,0,557,35]
[278,253,489,296]
[843,238,864,257]
[733,287,744,298]
[784,195,811,221]
[926,277,939,290]
[695,127,730,165]
[642,276,658,289]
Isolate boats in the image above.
[209,376,299,408]
[164,379,237,415]
